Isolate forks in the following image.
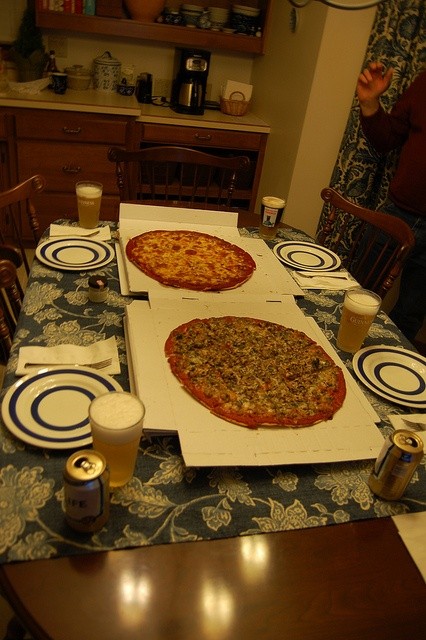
[293,269,350,283]
[399,416,426,431]
[25,358,116,370]
[48,230,103,238]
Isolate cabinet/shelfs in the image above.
[0,105,137,243]
[36,8,262,58]
[137,107,267,238]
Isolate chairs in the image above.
[0,174,44,280]
[320,188,415,305]
[1,261,25,364]
[106,146,251,212]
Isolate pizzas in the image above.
[127,229,255,291]
[164,315,347,429]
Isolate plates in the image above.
[0,365,124,449]
[351,346,426,410]
[36,236,116,274]
[272,240,341,274]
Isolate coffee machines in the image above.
[169,45,212,115]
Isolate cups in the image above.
[334,285,382,351]
[47,72,67,93]
[75,179,104,228]
[258,192,285,239]
[88,388,146,486]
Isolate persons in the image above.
[356,61,426,359]
